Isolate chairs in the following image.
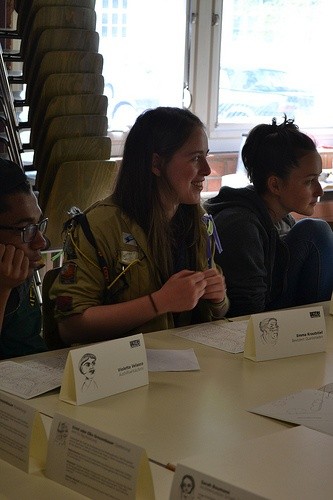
[0,0,122,286]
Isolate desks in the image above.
[0,301,333,500]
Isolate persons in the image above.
[0,160,52,362]
[49,108,228,348]
[202,112,333,319]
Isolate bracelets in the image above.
[149,292,158,317]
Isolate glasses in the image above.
[0,218,49,242]
